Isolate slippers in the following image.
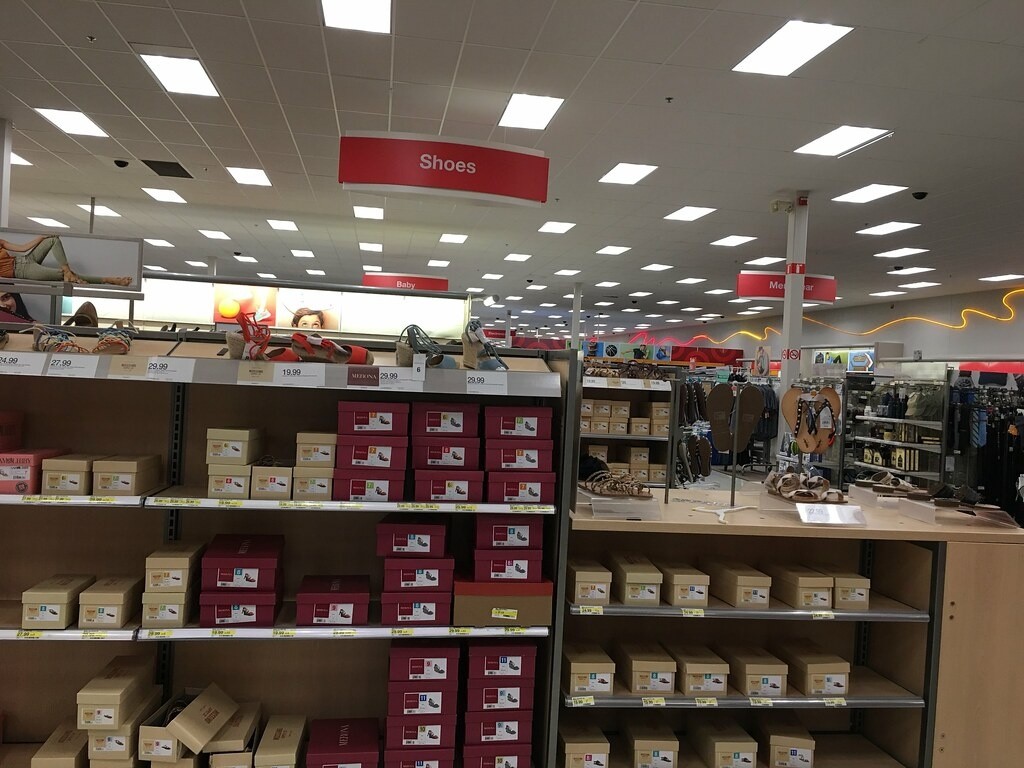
[907,482,958,501]
[934,484,1000,509]
[707,383,764,453]
[855,470,896,487]
[578,471,653,498]
[873,477,920,494]
[782,387,841,454]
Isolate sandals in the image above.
[765,463,849,504]
[19,324,89,353]
[92,320,139,355]
[111,277,132,286]
[64,269,89,284]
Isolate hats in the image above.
[905,391,941,417]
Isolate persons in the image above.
[290,308,325,330]
[247,286,271,321]
[586,342,598,356]
[656,346,670,361]
[0,292,42,335]
[825,352,841,364]
[0,234,133,287]
[621,344,649,359]
[754,347,769,376]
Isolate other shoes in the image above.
[75,302,98,327]
[0,329,9,349]
[679,381,712,484]
[585,360,674,381]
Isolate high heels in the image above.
[226,312,303,361]
[461,319,509,371]
[290,332,374,365]
[397,324,460,370]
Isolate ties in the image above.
[947,388,986,450]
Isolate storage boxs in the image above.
[558,710,816,768]
[200,534,285,627]
[378,513,553,627]
[205,426,337,501]
[307,717,379,768]
[581,399,671,437]
[141,540,208,629]
[77,574,145,629]
[21,573,95,629]
[296,575,370,626]
[562,637,851,697]
[567,552,871,611]
[334,401,556,504]
[30,655,308,768]
[0,411,161,496]
[581,445,666,483]
[384,639,537,768]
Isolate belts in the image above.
[991,391,1024,515]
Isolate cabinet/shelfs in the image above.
[0,321,579,768]
[577,362,680,489]
[838,382,950,491]
[557,490,1024,768]
